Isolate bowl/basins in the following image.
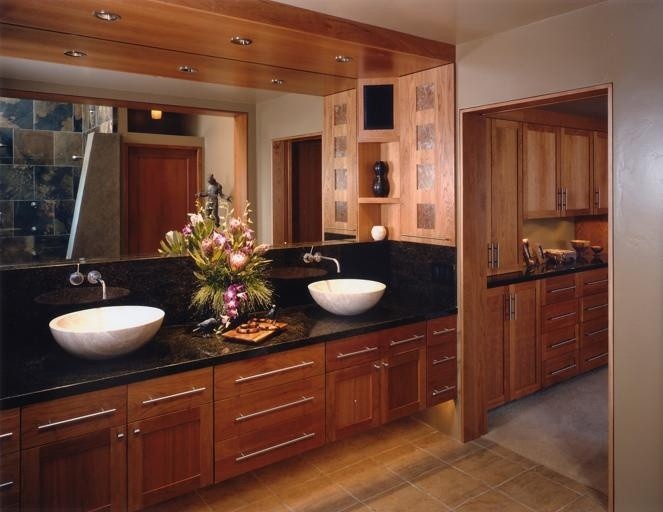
[591,246,604,254]
[570,240,591,253]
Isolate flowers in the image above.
[158,207,219,255]
[192,212,273,328]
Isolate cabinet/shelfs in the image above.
[541,273,578,388]
[523,121,593,219]
[214,342,325,484]
[20,366,214,512]
[0,405,21,512]
[593,130,608,216]
[487,117,523,276]
[426,314,457,407]
[577,266,608,375]
[487,280,541,410]
[325,321,427,444]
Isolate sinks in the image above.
[307,279,387,315]
[49,304,165,358]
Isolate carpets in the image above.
[480,363,607,496]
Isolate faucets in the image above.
[314,252,342,275]
[92,270,108,301]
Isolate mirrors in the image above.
[0,21,356,269]
